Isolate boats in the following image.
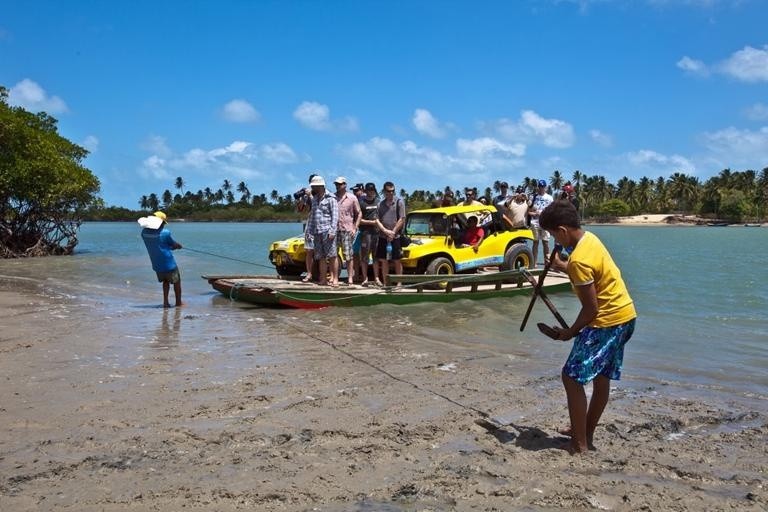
[201,262,573,309]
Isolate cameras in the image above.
[294,188,306,200]
[562,191,568,198]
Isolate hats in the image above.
[562,185,573,192]
[501,182,508,188]
[309,176,325,186]
[350,183,363,190]
[333,177,347,184]
[154,211,168,225]
[538,180,546,187]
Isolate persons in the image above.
[141,211,188,308]
[295,173,580,289]
[536,200,638,452]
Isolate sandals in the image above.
[302,274,384,288]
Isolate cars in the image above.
[268,232,347,276]
[353,204,535,290]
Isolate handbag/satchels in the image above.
[401,235,411,246]
